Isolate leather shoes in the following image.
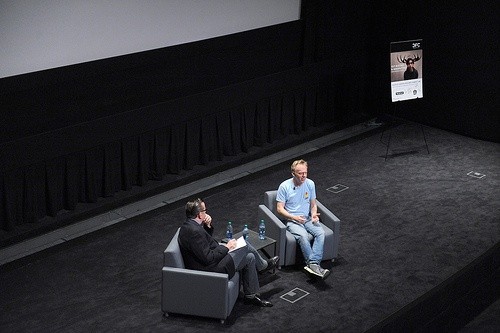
[259,256,280,275]
[243,295,273,307]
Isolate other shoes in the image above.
[319,266,330,279]
[304,264,324,280]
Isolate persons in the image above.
[178,197,280,308]
[403,58,419,81]
[277,160,331,279]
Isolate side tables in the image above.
[220,229,277,274]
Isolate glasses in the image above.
[196,208,207,214]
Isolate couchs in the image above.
[258,191,340,269]
[161,227,242,324]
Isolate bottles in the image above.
[226,221,233,241]
[258,220,266,240]
[242,225,248,241]
